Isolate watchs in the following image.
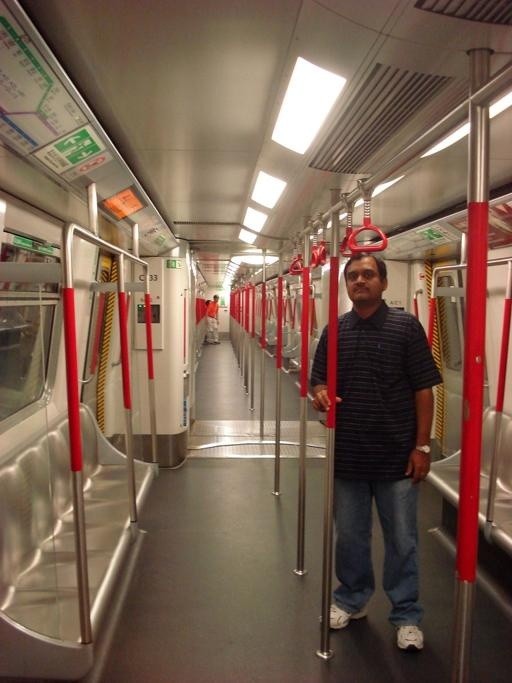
[416,444,432,454]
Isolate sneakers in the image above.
[396,622,426,652]
[319,597,369,629]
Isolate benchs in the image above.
[427,407,511,556]
[256,321,320,380]
[0,404,160,679]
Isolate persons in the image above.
[206,295,221,345]
[311,252,444,653]
